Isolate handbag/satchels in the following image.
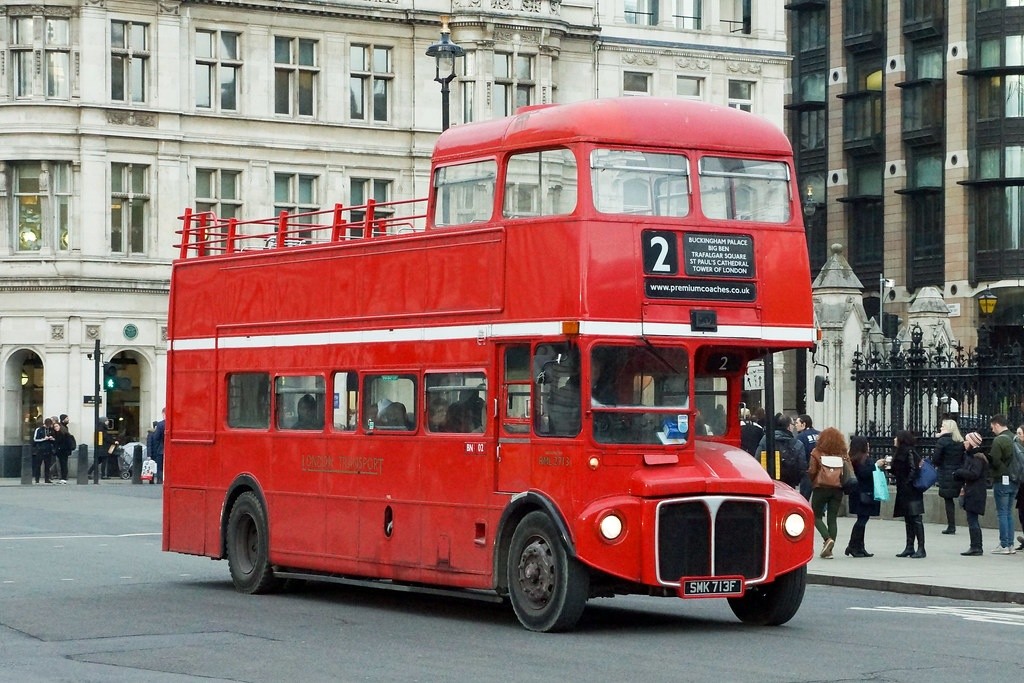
[141,460,157,481]
[841,452,858,495]
[872,463,890,502]
[908,449,938,492]
[860,491,877,505]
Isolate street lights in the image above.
[979,284,998,347]
[426,15,466,224]
[801,185,820,256]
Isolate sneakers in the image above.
[991,543,1010,554]
[1009,544,1015,553]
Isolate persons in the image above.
[951,431,990,556]
[33,414,76,484]
[426,390,485,433]
[987,414,1016,554]
[291,394,320,430]
[376,402,412,427]
[795,415,822,500]
[845,435,886,557]
[88,416,119,481]
[147,407,166,484]
[740,402,765,455]
[1015,425,1024,550]
[547,354,600,435]
[886,430,926,558]
[754,412,807,489]
[808,426,847,559]
[932,419,964,535]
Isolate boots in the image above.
[845,513,984,558]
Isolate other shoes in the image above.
[45,478,52,483]
[56,479,67,485]
[820,538,834,558]
[101,476,109,479]
[1014,541,1023,552]
[35,478,39,483]
[149,479,154,484]
[823,551,833,559]
[88,474,92,479]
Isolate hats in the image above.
[60,414,67,421]
[99,417,108,424]
[966,432,982,448]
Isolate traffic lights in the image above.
[104,364,118,389]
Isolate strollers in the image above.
[115,442,147,480]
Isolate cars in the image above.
[959,413,993,433]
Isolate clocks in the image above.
[123,324,138,340]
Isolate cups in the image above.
[884,456,893,469]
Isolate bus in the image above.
[162,96,830,632]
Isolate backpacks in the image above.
[976,451,995,489]
[997,433,1024,482]
[60,429,76,450]
[817,448,844,489]
[770,437,803,484]
